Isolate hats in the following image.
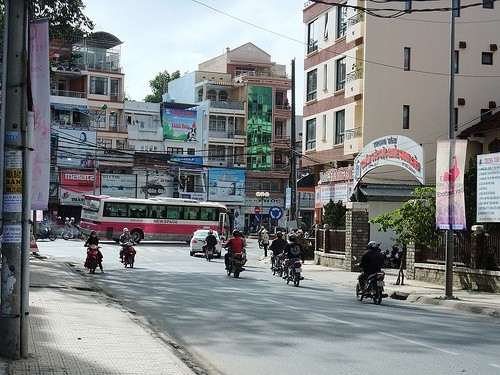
[91,230,96,235]
[277,231,281,236]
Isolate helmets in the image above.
[289,234,296,241]
[71,217,75,221]
[368,241,376,248]
[209,229,213,233]
[232,229,240,237]
[123,228,129,233]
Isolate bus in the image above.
[78,194,230,247]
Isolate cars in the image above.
[188,227,222,257]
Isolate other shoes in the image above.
[359,289,365,293]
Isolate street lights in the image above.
[71,103,115,196]
[256,191,270,229]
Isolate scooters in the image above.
[221,244,247,279]
[120,242,137,268]
[201,239,219,262]
[353,255,389,305]
[61,224,88,241]
[268,245,305,287]
[84,244,102,274]
[37,224,58,241]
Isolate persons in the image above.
[261,229,269,256]
[357,241,385,294]
[258,226,264,247]
[268,231,288,269]
[278,234,304,280]
[239,231,247,260]
[84,230,104,273]
[202,229,218,258]
[119,228,136,263]
[39,217,80,236]
[286,227,308,263]
[223,230,244,270]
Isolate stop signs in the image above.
[254,206,260,215]
